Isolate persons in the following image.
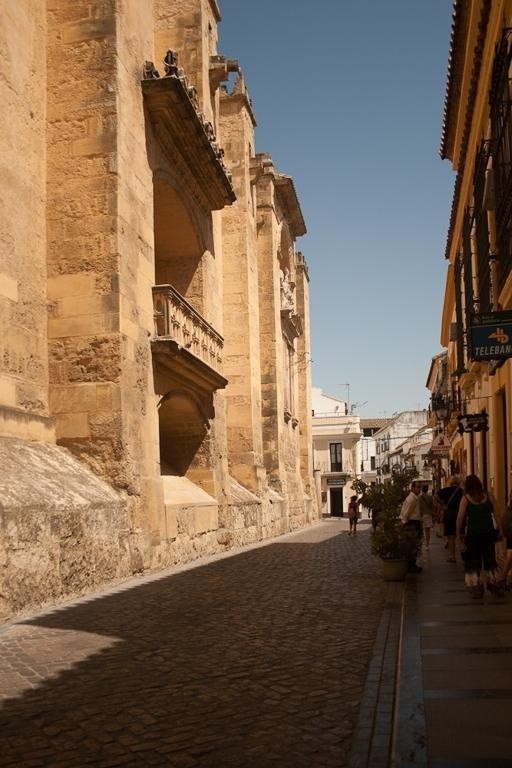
[398,474,511,600]
[348,495,359,536]
[368,493,385,533]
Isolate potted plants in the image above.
[369,508,423,581]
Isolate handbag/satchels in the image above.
[438,509,448,524]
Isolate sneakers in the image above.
[407,564,423,574]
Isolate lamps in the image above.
[429,392,493,421]
